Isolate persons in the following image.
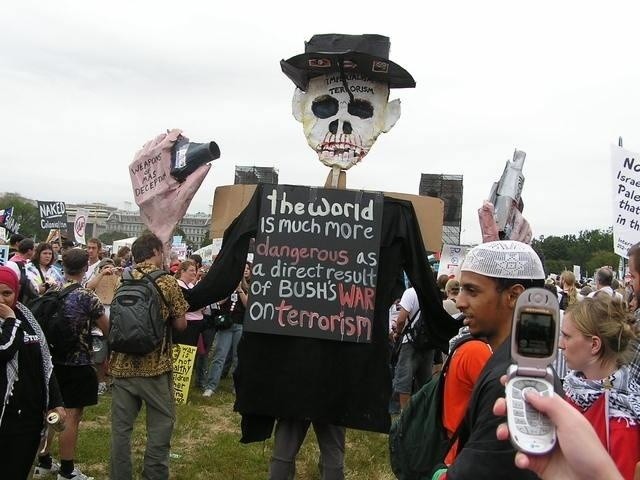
[128,32,536,445]
[380,236,639,480]
[262,412,350,480]
[1,234,257,479]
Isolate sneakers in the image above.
[33,458,94,480]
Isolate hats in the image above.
[461,240,545,279]
[99,258,115,267]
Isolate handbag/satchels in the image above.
[215,313,233,330]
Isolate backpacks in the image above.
[109,270,167,354]
[403,309,438,349]
[29,283,82,346]
[389,336,488,480]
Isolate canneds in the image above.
[45,412,65,433]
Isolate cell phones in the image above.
[504,288,560,458]
